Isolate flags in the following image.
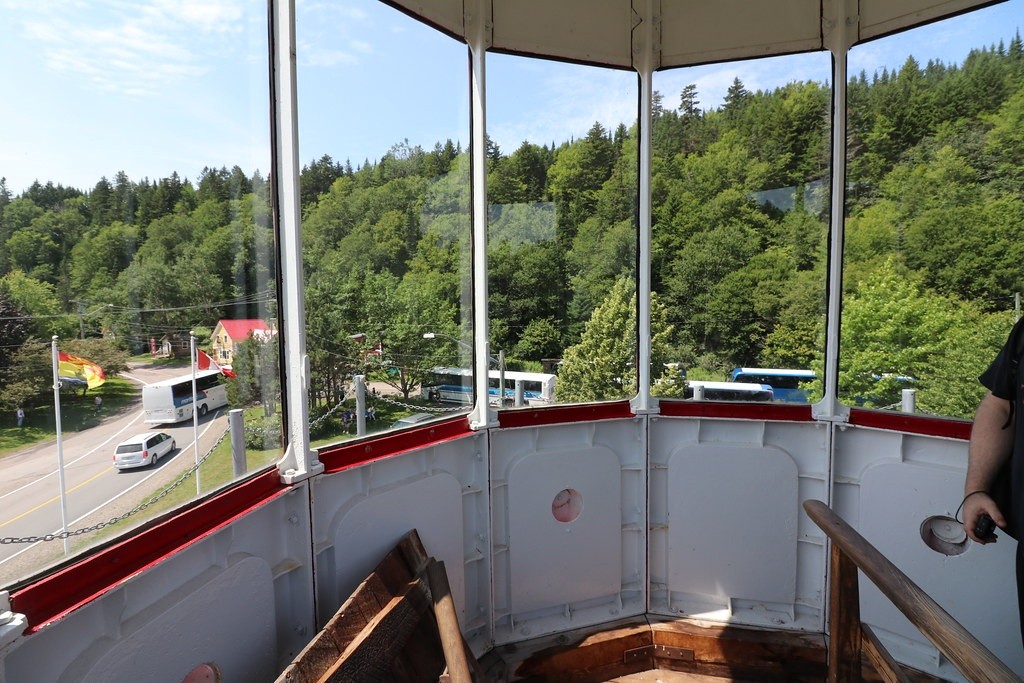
[196,349,237,379]
[58,351,106,390]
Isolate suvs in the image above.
[155,354,173,361]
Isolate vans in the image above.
[112,432,177,470]
[387,413,435,428]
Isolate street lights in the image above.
[361,350,367,368]
[424,333,505,408]
[79,304,113,340]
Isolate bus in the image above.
[654,380,774,404]
[141,364,237,424]
[552,362,686,383]
[729,368,916,411]
[421,364,559,408]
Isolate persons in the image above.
[963,315,1024,649]
[94,394,102,413]
[16,406,25,428]
[336,384,376,434]
